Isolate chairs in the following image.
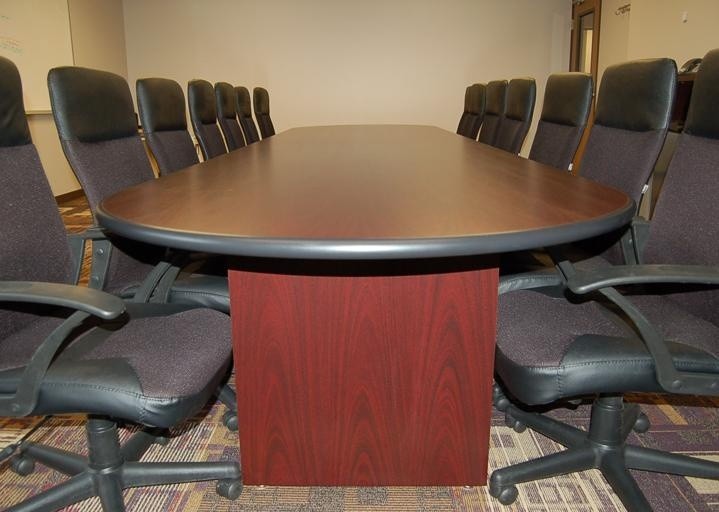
[454,57,718,433]
[489,50,719,511]
[1,54,244,511]
[46,66,276,446]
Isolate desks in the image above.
[94,124,637,486]
